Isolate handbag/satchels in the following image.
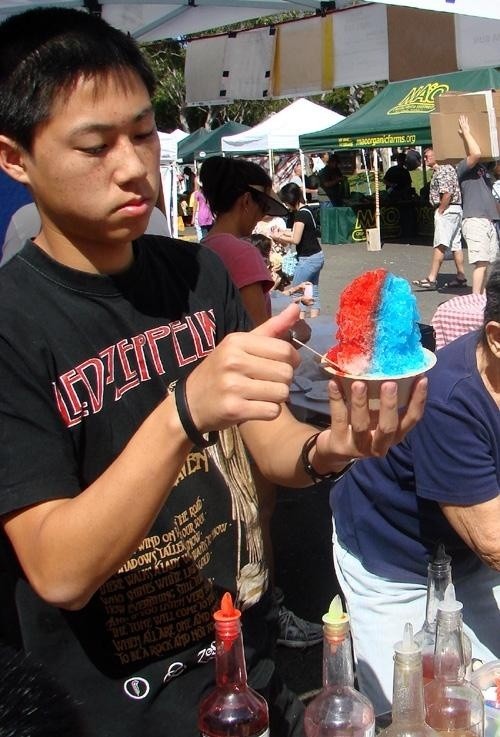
[282,252,300,277]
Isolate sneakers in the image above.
[278,605,323,649]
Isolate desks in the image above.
[320,202,413,244]
[412,202,435,235]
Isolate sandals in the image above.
[441,277,467,288]
[412,278,438,290]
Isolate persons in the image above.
[179,197,191,226]
[188,191,202,243]
[250,214,292,258]
[329,260,500,725]
[250,234,316,306]
[383,153,412,190]
[312,152,329,173]
[0,202,174,262]
[199,155,312,350]
[290,160,318,193]
[191,186,216,238]
[268,183,324,320]
[183,166,197,226]
[412,147,467,289]
[455,114,499,293]
[0,6,430,737]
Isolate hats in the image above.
[247,183,290,217]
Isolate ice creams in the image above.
[320,267,437,412]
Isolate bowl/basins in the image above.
[321,348,438,413]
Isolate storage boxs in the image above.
[429,108,499,159]
[438,90,494,113]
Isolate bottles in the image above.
[197,594,270,737]
[303,595,376,737]
[379,541,500,737]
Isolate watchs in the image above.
[289,329,299,340]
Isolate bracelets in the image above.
[175,370,218,448]
[289,291,291,295]
[303,431,357,485]
